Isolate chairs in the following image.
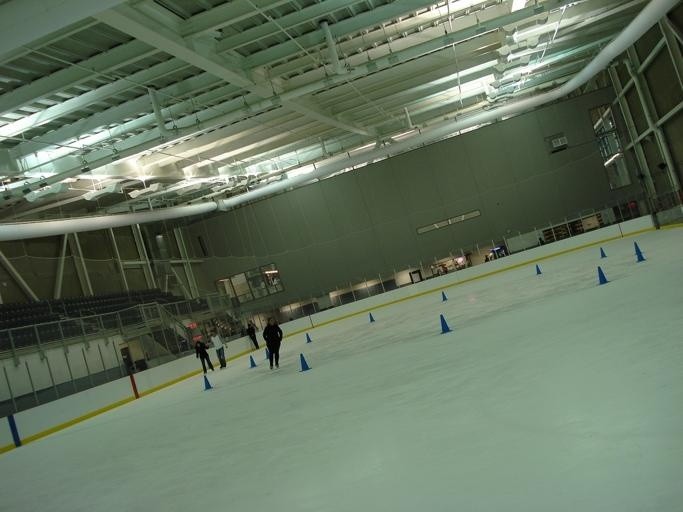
[1,288,212,353]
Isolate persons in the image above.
[434,236,555,278]
[262,315,282,370]
[245,322,259,351]
[208,329,229,370]
[192,340,214,375]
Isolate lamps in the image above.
[482,12,551,110]
[21,172,252,202]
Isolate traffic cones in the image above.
[305,332,313,343]
[535,264,542,276]
[298,353,313,372]
[369,312,375,323]
[265,349,269,359]
[203,375,213,392]
[249,355,258,369]
[438,314,453,334]
[441,291,448,302]
[633,241,647,263]
[596,266,610,286]
[599,247,609,259]
